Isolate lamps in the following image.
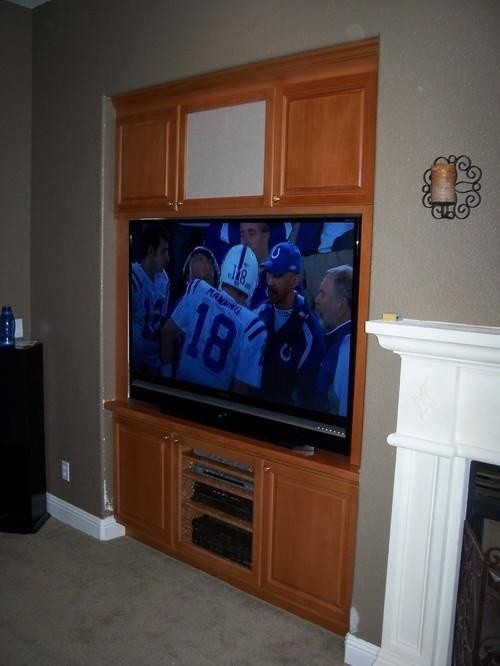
[420,153,483,221]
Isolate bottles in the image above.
[0,303,17,349]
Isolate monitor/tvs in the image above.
[127,213,364,457]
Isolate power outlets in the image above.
[14,318,24,339]
[61,461,70,482]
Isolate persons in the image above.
[131,223,354,418]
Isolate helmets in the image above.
[219,244,259,298]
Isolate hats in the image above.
[259,242,302,274]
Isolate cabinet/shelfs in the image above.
[0,342,51,535]
[103,32,379,639]
